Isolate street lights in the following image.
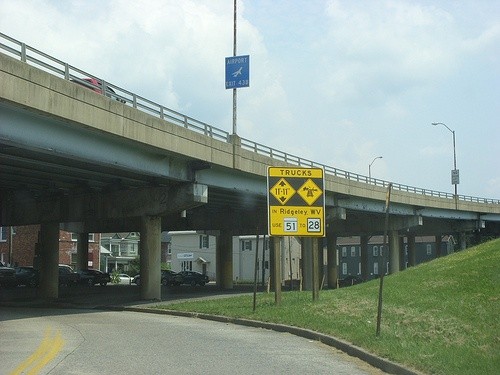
[432,123,459,211]
[369,156,383,184]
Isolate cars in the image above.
[119,274,134,283]
[131,270,185,287]
[74,268,111,286]
[58,264,80,288]
[177,270,210,286]
[0,262,17,284]
[16,266,33,285]
[70,79,126,104]
